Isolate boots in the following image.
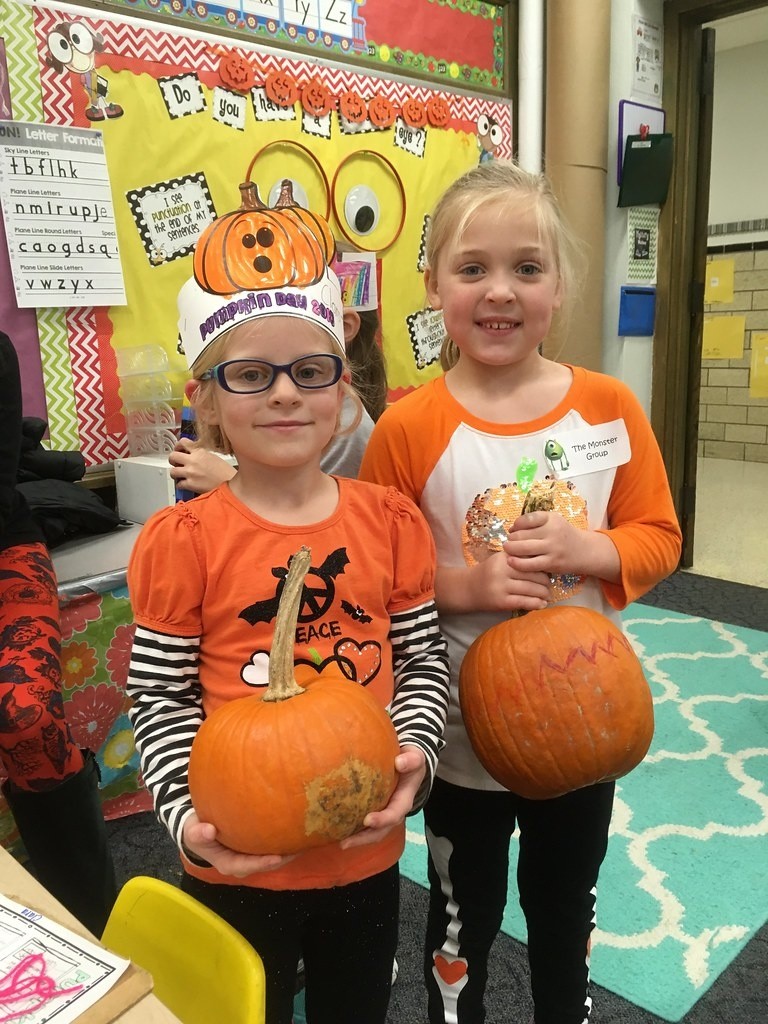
[1,748,117,941]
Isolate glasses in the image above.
[197,353,344,395]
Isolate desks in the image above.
[0,844,181,1024]
[53,519,154,819]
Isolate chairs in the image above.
[100,875,266,1023]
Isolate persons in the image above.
[1,326,116,943]
[169,254,376,495]
[125,182,447,1024]
[356,160,683,1024]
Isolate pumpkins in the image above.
[459,493,656,801]
[188,545,400,857]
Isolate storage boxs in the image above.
[114,449,238,526]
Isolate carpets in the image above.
[105,570,768,1024]
